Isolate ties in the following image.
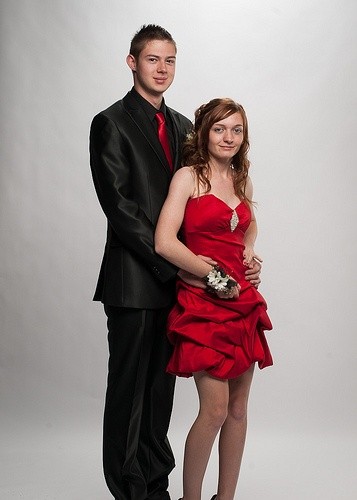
[155,112,174,171]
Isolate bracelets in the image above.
[203,265,237,294]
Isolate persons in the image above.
[156,98,264,500]
[89,23,265,500]
